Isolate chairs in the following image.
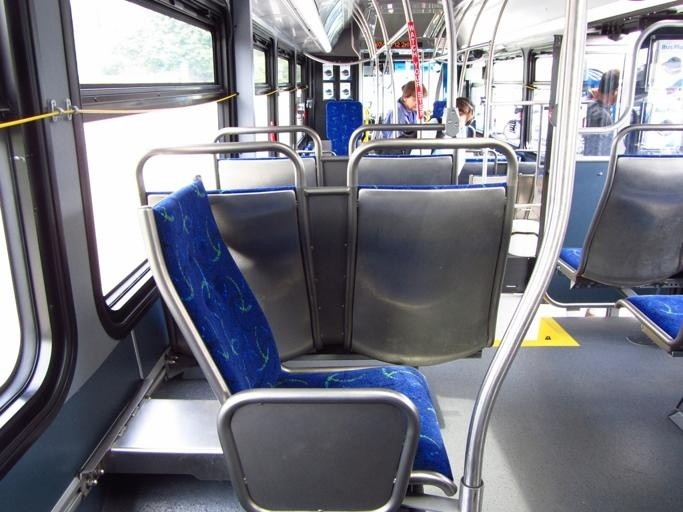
[135,141,325,368]
[549,125,681,289]
[339,136,519,368]
[348,124,459,187]
[210,125,325,187]
[463,124,541,220]
[615,295,682,430]
[141,174,460,510]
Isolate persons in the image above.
[583,69,621,157]
[430,96,476,176]
[372,80,428,156]
